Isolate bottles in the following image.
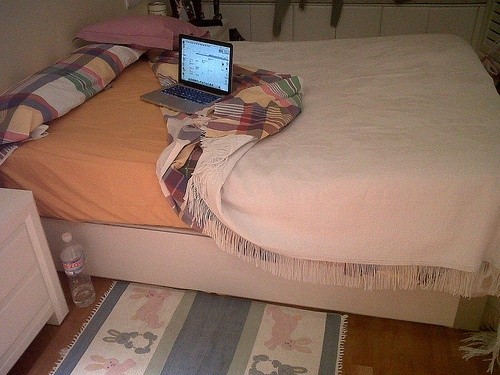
[60,232,96,308]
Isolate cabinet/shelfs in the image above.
[479,0,500,88]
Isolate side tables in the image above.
[200,1,489,49]
[199,18,230,40]
[0,188,70,375]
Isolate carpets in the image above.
[47,281,346,375]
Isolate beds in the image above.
[0,32,500,332]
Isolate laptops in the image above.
[140,33,233,114]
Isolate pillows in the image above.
[73,15,209,51]
[0,44,143,144]
